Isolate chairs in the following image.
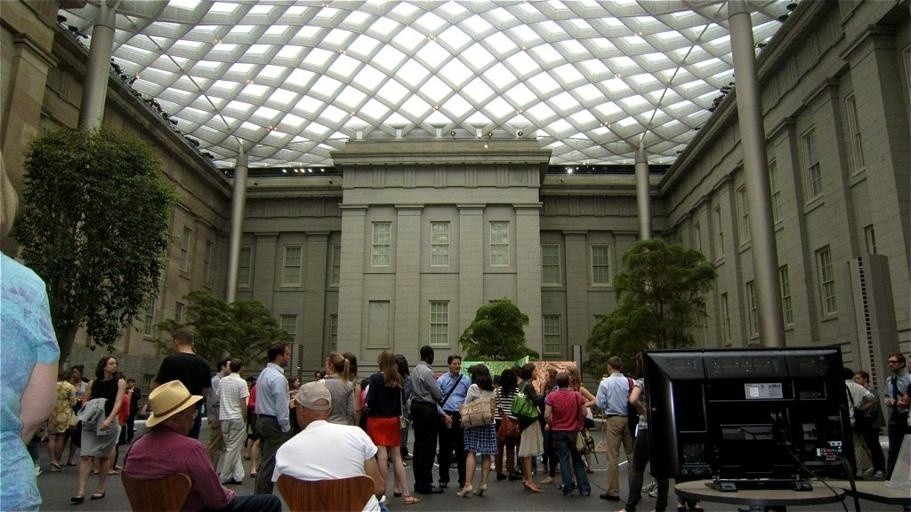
[277,474,377,512]
[121,470,193,512]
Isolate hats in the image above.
[142,379,205,429]
[292,380,333,413]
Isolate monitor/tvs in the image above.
[640,346,857,492]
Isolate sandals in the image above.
[90,490,106,502]
[69,493,84,504]
[402,493,422,506]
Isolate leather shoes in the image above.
[412,483,445,496]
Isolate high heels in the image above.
[472,483,488,497]
[523,479,543,495]
[454,484,473,499]
[48,461,64,473]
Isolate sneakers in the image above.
[539,476,556,485]
[220,475,243,487]
[598,491,621,504]
[495,474,523,484]
[641,481,659,498]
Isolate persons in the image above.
[842,354,911,481]
[1,155,60,512]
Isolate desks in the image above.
[673,478,846,512]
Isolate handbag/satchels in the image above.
[458,383,496,428]
[495,412,521,446]
[397,415,410,430]
[511,382,540,419]
[574,430,595,456]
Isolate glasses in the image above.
[185,410,198,420]
